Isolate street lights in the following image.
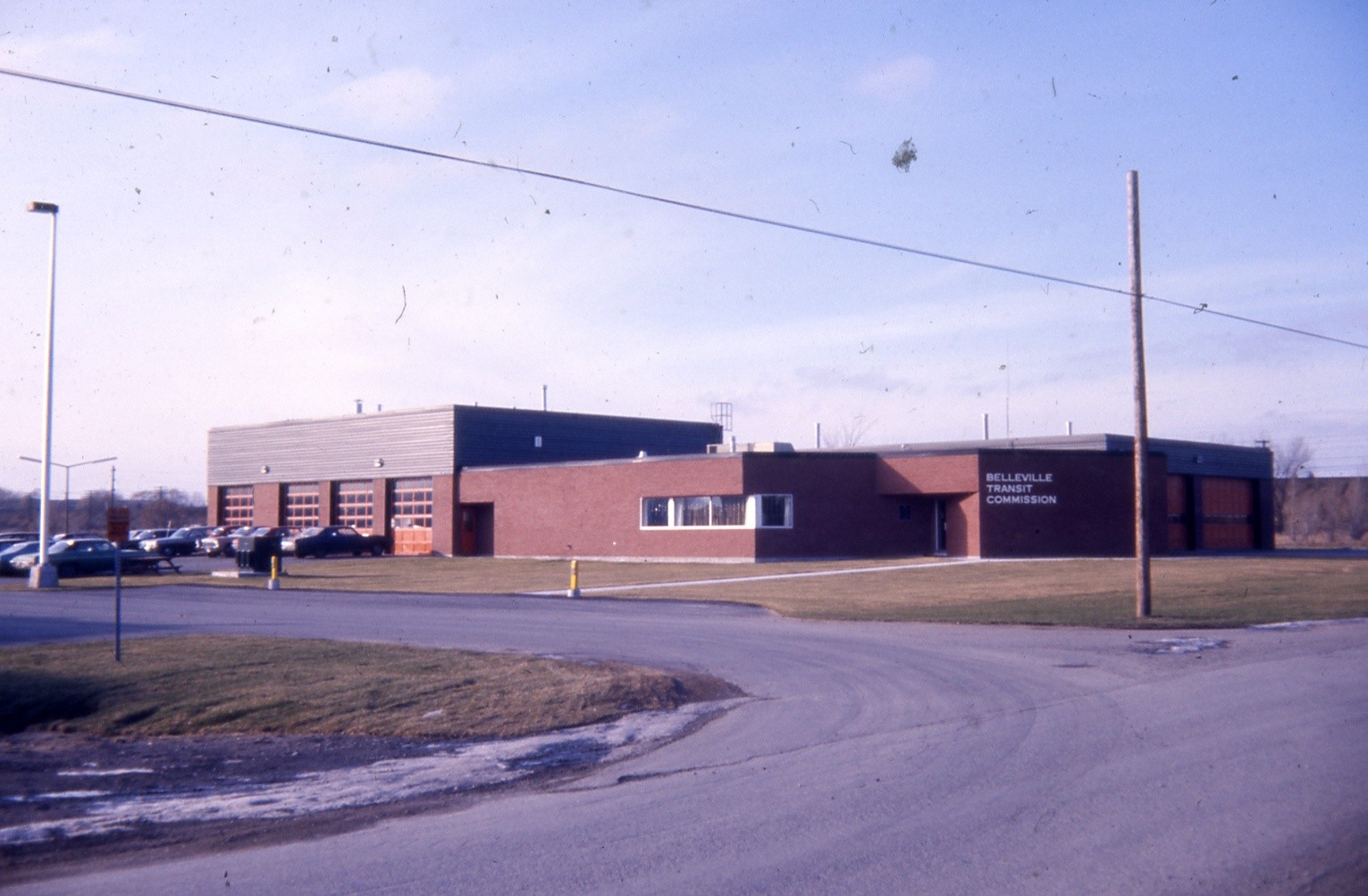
[26,199,62,588]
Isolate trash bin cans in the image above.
[236,536,283,572]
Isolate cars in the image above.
[0,529,110,576]
[111,524,302,561]
[281,525,392,559]
[9,537,158,576]
